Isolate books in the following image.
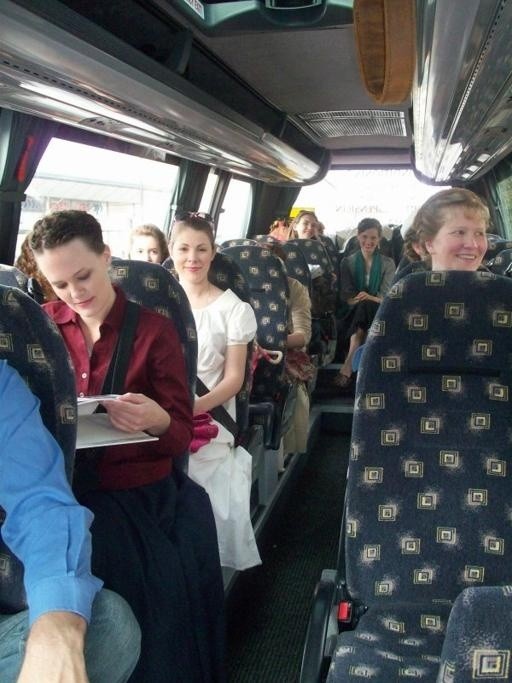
[72,393,162,450]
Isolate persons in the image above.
[332,216,397,396]
[1,357,142,682]
[415,187,490,272]
[127,223,167,266]
[255,209,337,476]
[403,223,429,262]
[16,234,60,302]
[157,213,263,572]
[31,210,226,681]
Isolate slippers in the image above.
[333,372,351,386]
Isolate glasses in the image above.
[167,211,215,243]
